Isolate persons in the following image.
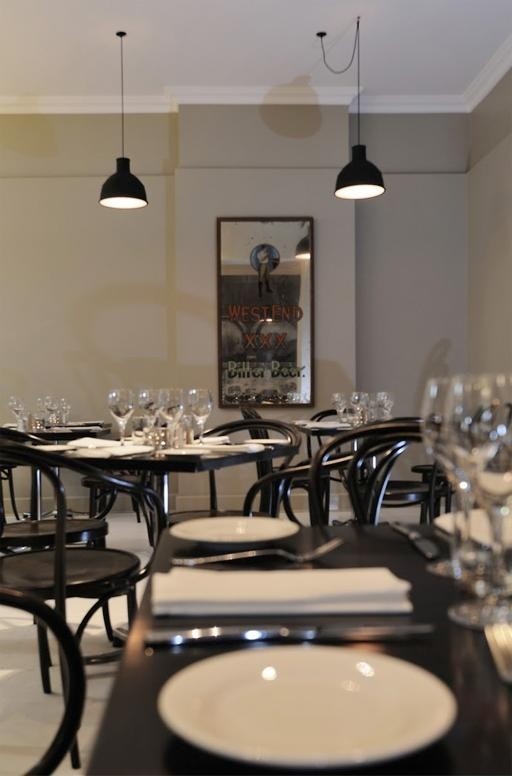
[256,244,274,296]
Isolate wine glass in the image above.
[159,387,184,449]
[187,388,211,446]
[5,391,75,436]
[332,389,394,429]
[137,389,161,441]
[110,390,132,449]
[419,377,510,630]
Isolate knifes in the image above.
[141,623,435,647]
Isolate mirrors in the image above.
[217,215,315,409]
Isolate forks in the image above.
[167,537,346,564]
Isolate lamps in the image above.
[97,30,149,208]
[295,235,311,260]
[317,18,386,200]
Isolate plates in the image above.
[157,642,459,770]
[169,516,301,551]
[30,438,292,463]
